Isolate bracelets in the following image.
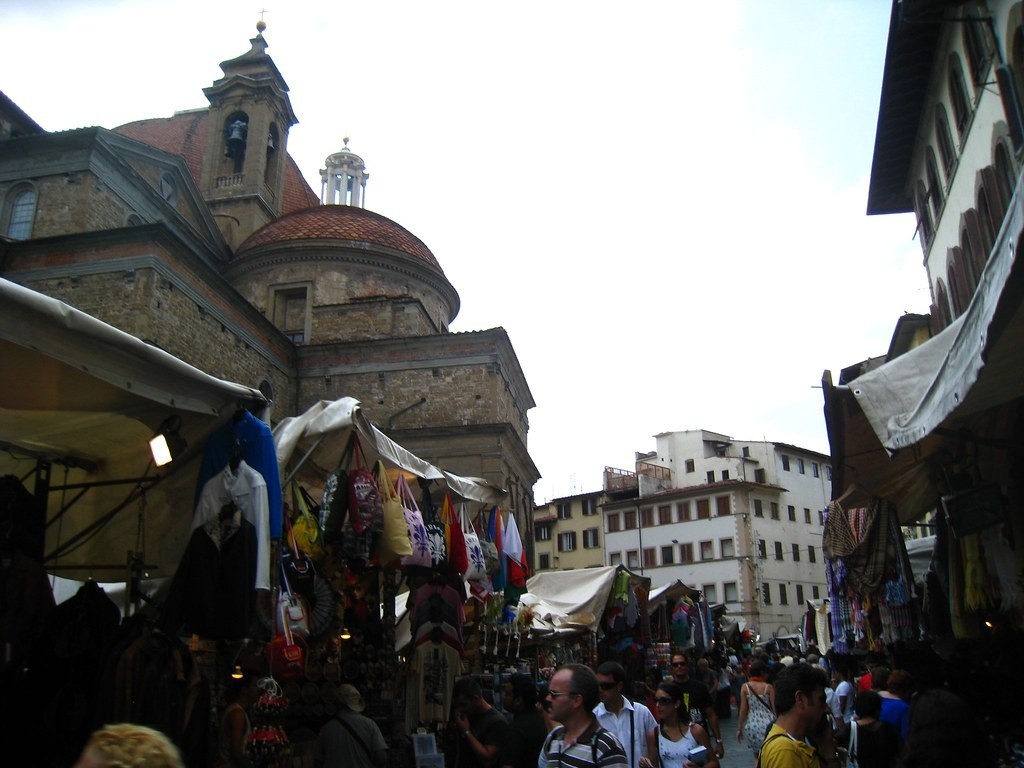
[464,730,470,734]
[716,739,722,743]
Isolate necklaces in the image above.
[750,677,764,680]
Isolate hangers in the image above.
[428,584,443,601]
[430,634,442,644]
[232,399,246,418]
[431,613,443,624]
[430,624,444,634]
[228,440,242,464]
[837,467,872,508]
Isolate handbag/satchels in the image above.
[373,460,412,568]
[348,432,385,537]
[397,474,433,568]
[267,464,325,678]
[476,507,500,577]
[318,431,353,535]
[457,502,486,582]
[440,492,468,574]
[846,720,859,768]
[421,484,448,568]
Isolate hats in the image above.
[333,683,366,713]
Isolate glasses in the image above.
[671,662,686,667]
[653,696,673,705]
[549,691,570,698]
[599,682,618,690]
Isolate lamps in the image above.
[149,414,188,468]
[941,473,1006,540]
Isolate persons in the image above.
[79,640,999,768]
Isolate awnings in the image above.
[2,277,702,639]
[821,159,1024,525]
